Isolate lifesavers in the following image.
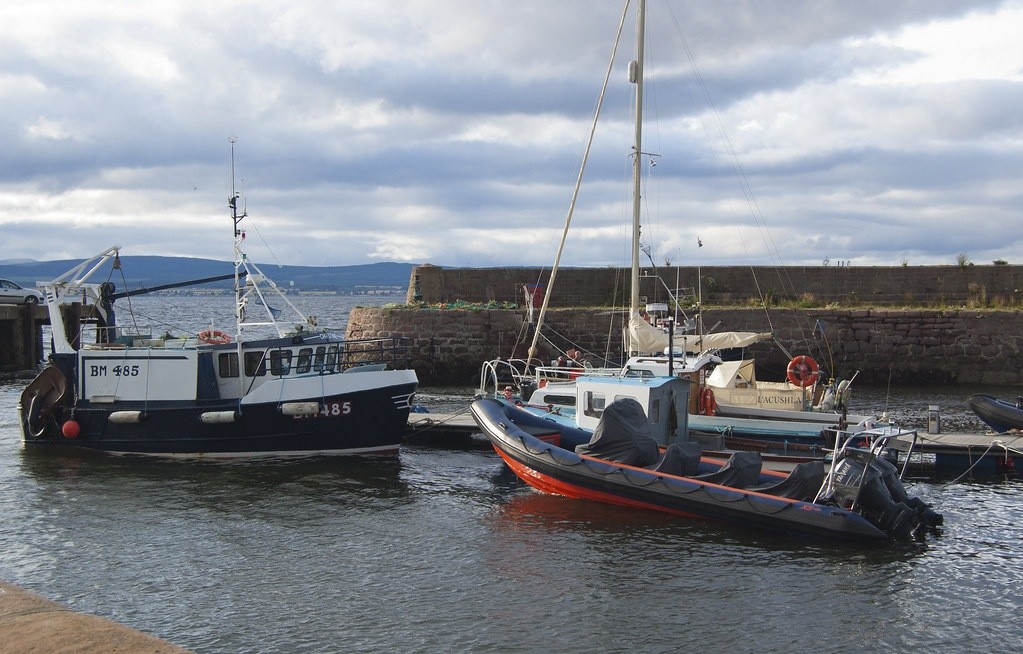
[699,387,717,416]
[787,355,819,387]
[199,330,231,345]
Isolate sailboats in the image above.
[471,0,918,437]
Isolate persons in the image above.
[571,351,584,368]
[556,356,567,378]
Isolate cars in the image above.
[0,279,45,307]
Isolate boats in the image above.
[968,392,1022,433]
[18,134,421,462]
[470,378,945,546]
